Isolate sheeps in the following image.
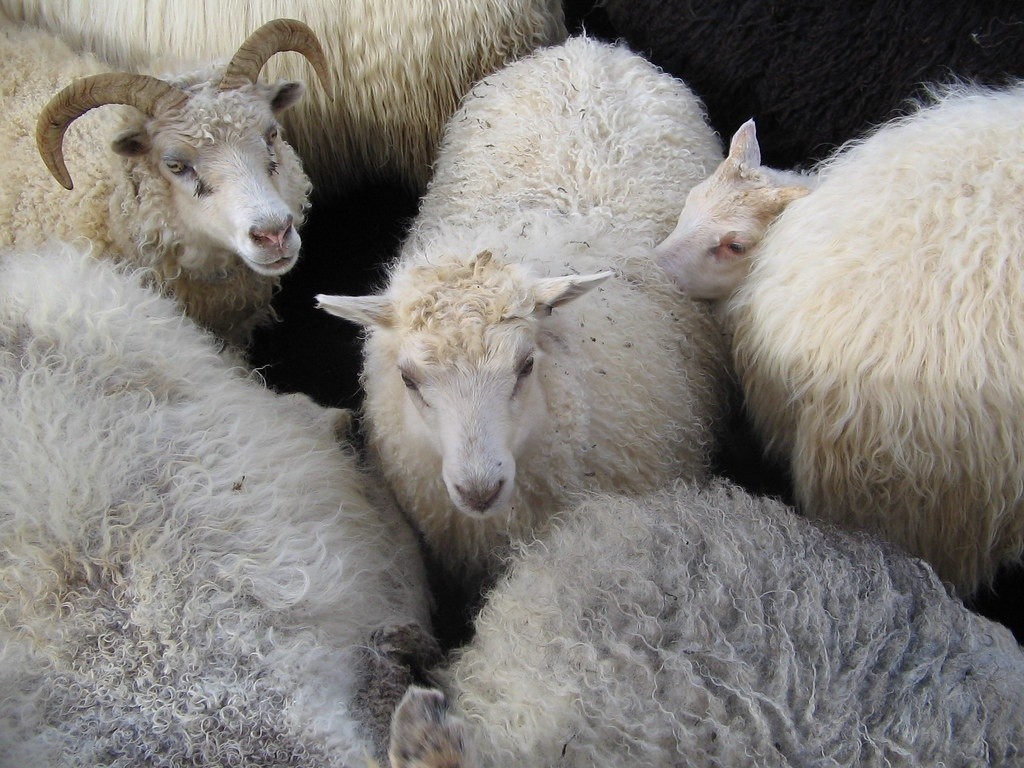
[0,0,1021,764]
